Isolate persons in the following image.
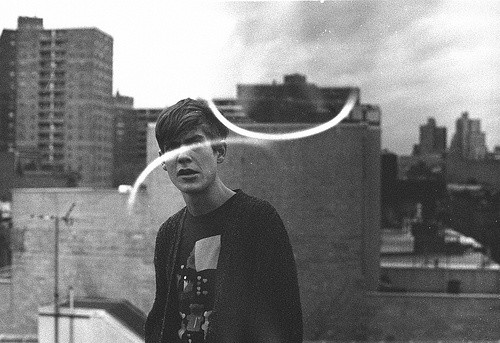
[142,96,306,343]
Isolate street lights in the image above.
[30,212,74,343]
